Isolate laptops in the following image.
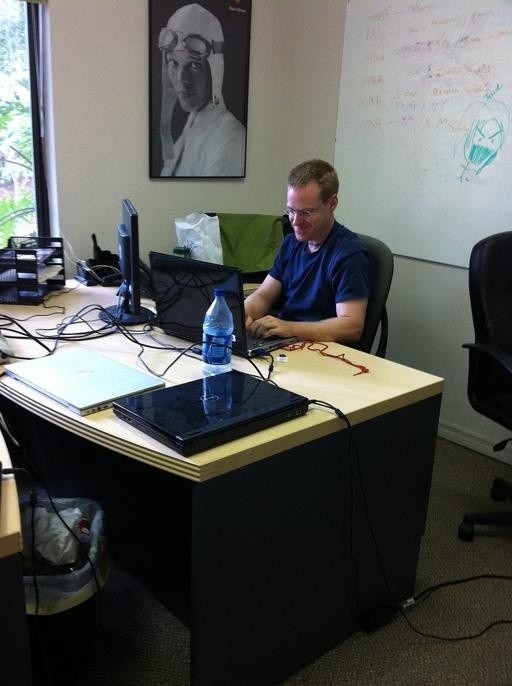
[148,251,299,358]
[3,349,165,416]
[113,369,309,457]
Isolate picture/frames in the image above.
[148,0,253,179]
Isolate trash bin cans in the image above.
[20,498,109,686]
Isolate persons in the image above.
[244,160,370,342]
[159,3,246,177]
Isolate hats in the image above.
[159,3,225,107]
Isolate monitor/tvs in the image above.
[98,200,155,325]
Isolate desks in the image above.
[0,275,448,686]
[0,428,34,686]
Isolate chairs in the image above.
[174,213,288,284]
[458,231,512,541]
[270,232,394,358]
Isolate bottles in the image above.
[202,288,234,375]
[204,375,232,425]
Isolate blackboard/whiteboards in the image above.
[331,0,512,269]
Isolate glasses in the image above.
[157,28,223,58]
[285,197,329,217]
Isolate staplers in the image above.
[102,274,122,287]
